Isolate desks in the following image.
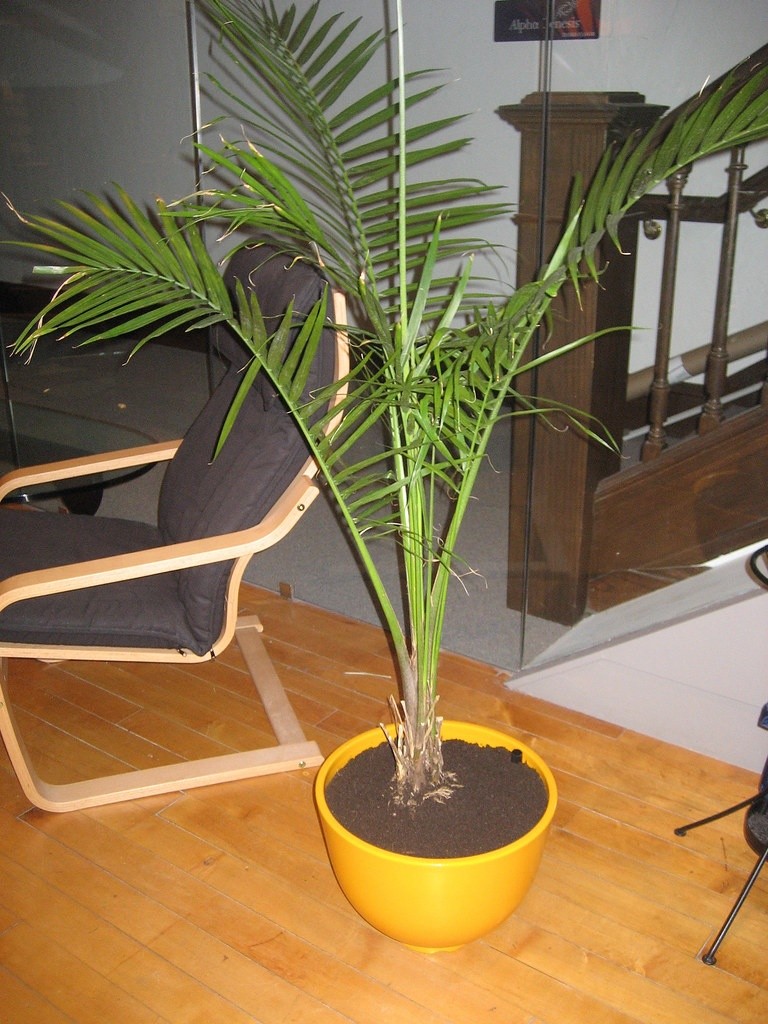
[0,322,233,517]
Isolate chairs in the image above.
[0,234,350,814]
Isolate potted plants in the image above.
[0,0,768,954]
